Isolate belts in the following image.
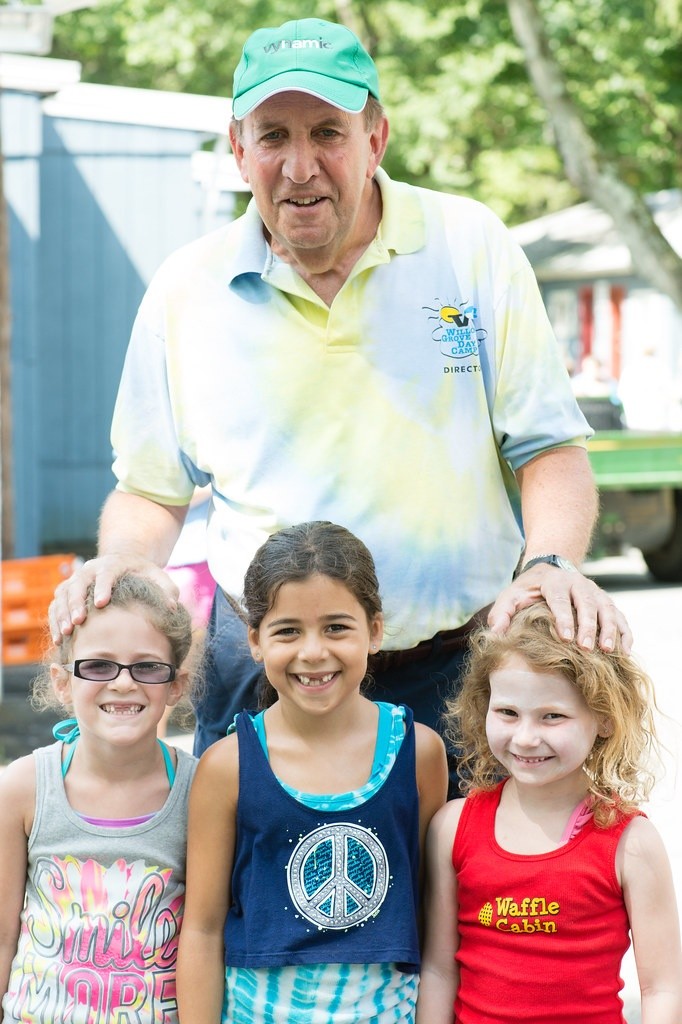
[221,589,493,667]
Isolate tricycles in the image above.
[499,389,682,585]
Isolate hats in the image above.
[232,18,380,121]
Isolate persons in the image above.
[49,18,633,804]
[416,603,682,1024]
[176,520,449,1024]
[1,575,200,1024]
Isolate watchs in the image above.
[522,554,577,573]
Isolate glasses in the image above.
[63,659,177,684]
